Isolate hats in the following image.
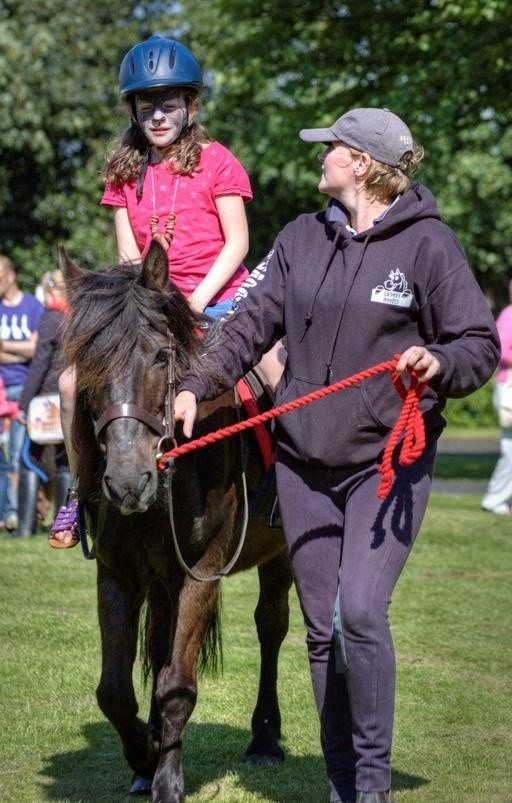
[300,107,414,172]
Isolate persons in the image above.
[0,377,28,531]
[0,255,45,534]
[11,269,73,534]
[482,278,511,516]
[46,33,285,552]
[177,107,503,802]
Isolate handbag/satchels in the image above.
[27,394,65,444]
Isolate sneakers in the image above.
[480,498,510,515]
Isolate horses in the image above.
[55,239,297,802]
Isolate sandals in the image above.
[48,501,84,549]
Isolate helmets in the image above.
[119,35,202,95]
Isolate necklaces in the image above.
[141,147,195,254]
